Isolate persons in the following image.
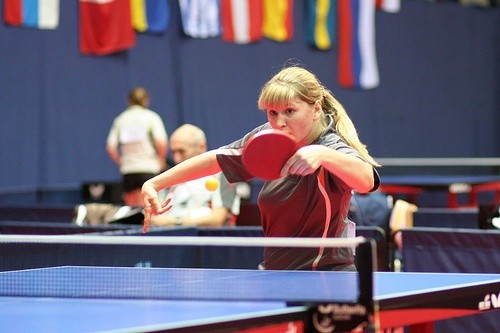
[105,87,237,228]
[139,67,382,272]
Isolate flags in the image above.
[2,0,402,90]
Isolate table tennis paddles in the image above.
[241,129,322,181]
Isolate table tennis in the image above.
[205,178,219,192]
